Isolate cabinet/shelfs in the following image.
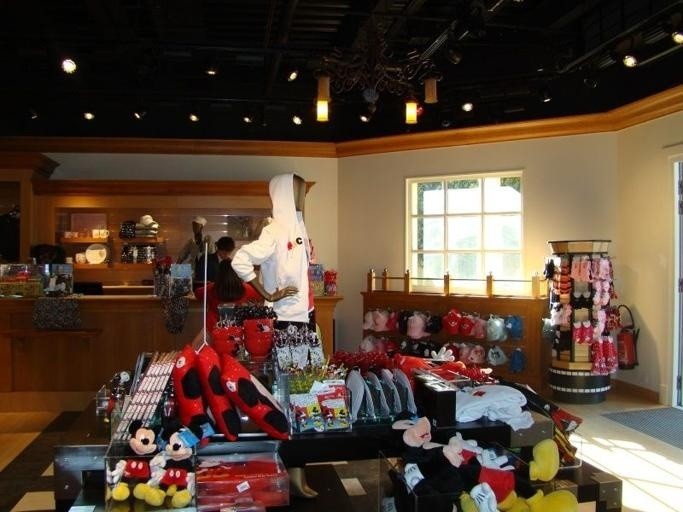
[58,236,164,271]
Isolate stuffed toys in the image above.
[144,420,203,509]
[108,420,162,502]
[374,410,579,511]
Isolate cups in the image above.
[91,228,110,239]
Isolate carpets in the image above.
[600,406,683,449]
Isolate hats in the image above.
[359,310,526,373]
[192,216,207,227]
[140,215,156,226]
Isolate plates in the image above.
[84,244,108,264]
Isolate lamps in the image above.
[671,20,683,44]
[312,12,444,125]
[622,48,640,69]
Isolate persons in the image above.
[192,237,235,293]
[230,173,318,344]
[175,215,217,280]
[192,256,265,356]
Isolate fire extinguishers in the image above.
[616,305,640,370]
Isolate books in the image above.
[112,348,181,441]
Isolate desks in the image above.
[53,356,622,510]
